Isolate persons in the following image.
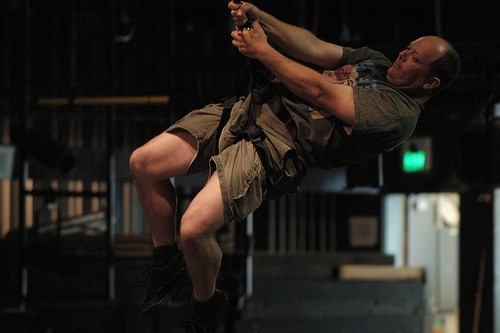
[129,1,458,333]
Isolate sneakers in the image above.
[139,254,190,316]
[184,285,229,333]
